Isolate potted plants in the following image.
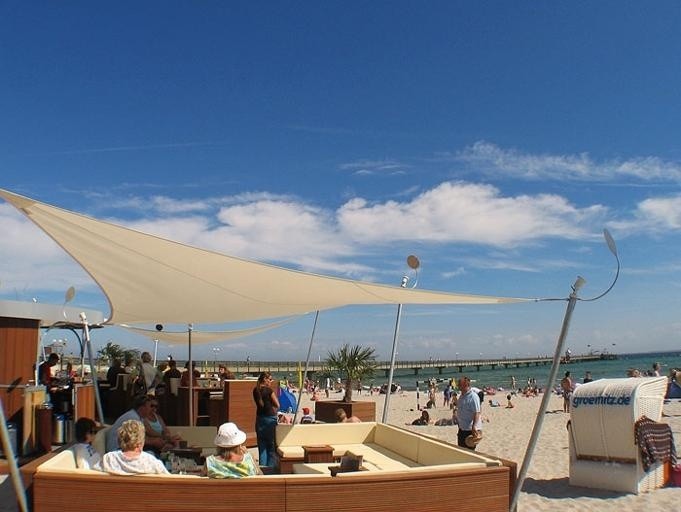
[310,344,383,421]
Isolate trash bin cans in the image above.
[53,419,74,445]
[0,424,18,458]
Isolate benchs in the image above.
[32,420,519,512]
[108,372,278,434]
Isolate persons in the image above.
[202,422,260,478]
[336,408,362,424]
[456,376,482,450]
[218,363,235,380]
[252,371,280,471]
[246,356,251,366]
[300,407,312,424]
[406,361,680,426]
[38,352,200,477]
[283,376,379,401]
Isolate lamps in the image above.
[61,286,86,328]
[398,251,420,291]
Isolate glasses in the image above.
[86,431,98,434]
[150,404,159,408]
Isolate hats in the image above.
[214,422,247,448]
[463,430,483,448]
